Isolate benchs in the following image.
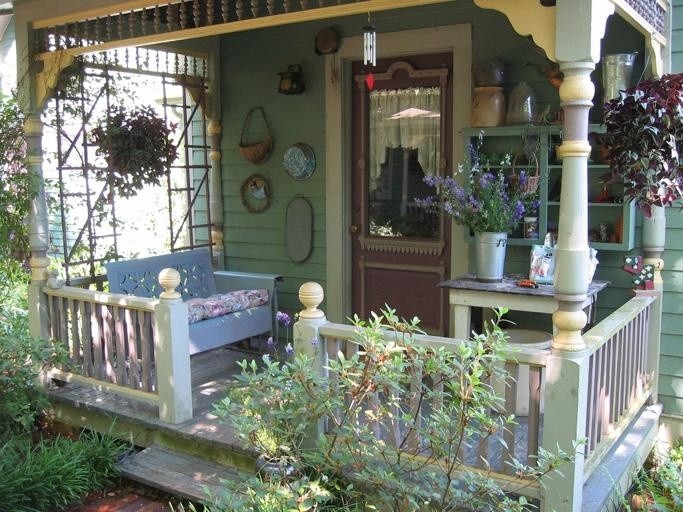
[102,247,284,369]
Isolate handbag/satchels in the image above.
[529,231,599,284]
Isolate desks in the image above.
[435,273,612,342]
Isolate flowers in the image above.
[414,129,553,232]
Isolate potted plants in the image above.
[209,353,351,484]
[84,102,179,202]
[595,71,683,220]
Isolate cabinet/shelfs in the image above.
[463,118,640,253]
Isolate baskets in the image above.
[508,148,540,194]
[238,106,273,162]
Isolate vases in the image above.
[470,85,506,128]
[474,230,509,284]
